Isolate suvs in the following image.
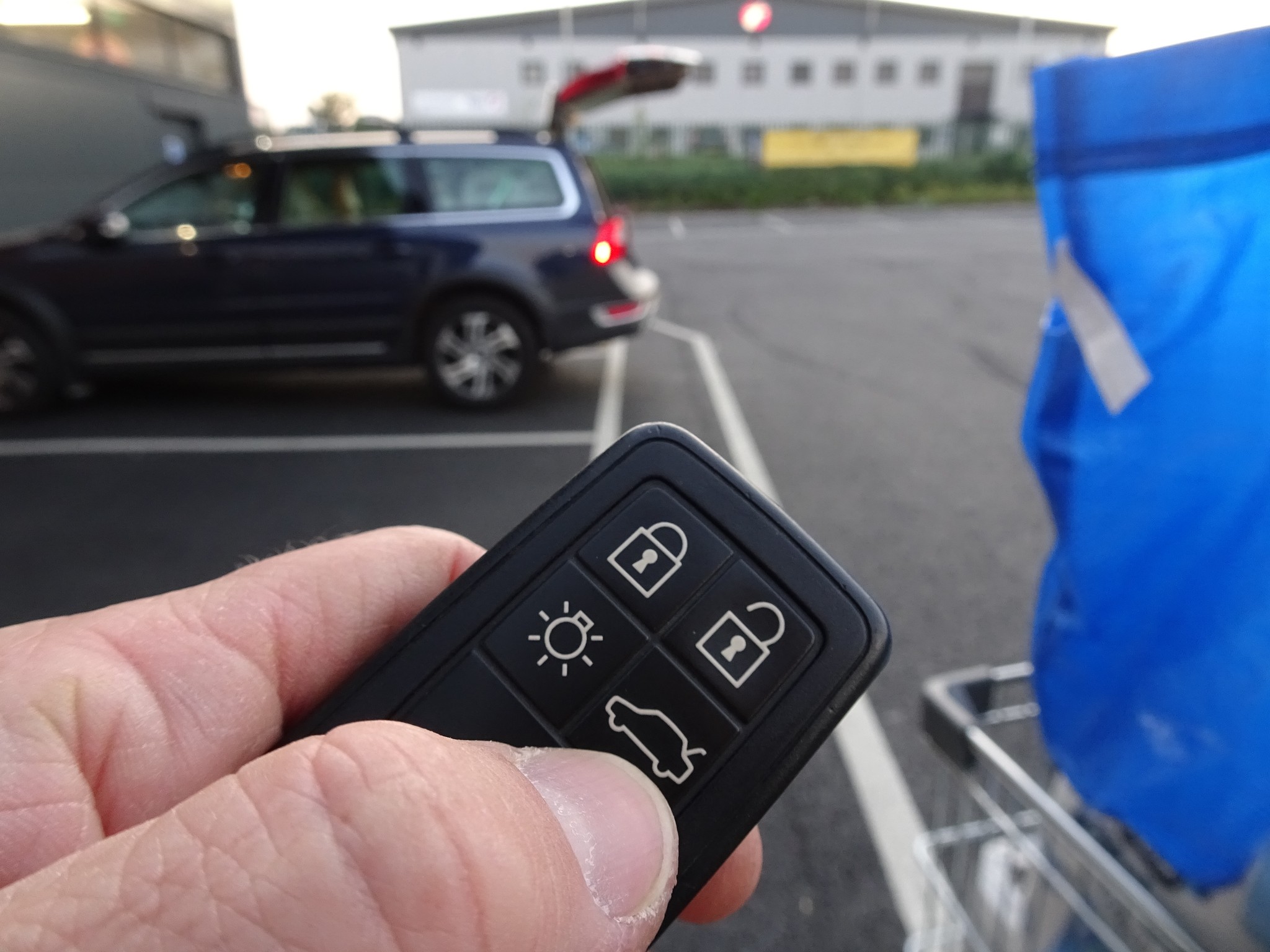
[0,46,700,422]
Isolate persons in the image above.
[0,524,765,952]
